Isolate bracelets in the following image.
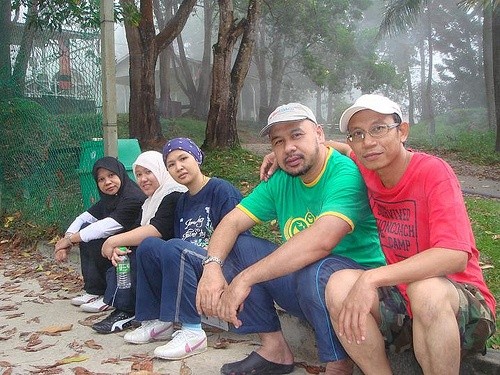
[68,236,78,245]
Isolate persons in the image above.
[91,150,188,334]
[260,93,496,375]
[195,102,387,375]
[55,157,149,313]
[124,138,251,360]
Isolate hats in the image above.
[162,137,204,166]
[258,103,317,136]
[339,94,403,133]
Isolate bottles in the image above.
[116,247,131,289]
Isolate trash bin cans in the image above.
[77,138,141,211]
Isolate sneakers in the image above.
[123,318,173,344]
[91,308,141,333]
[70,293,99,306]
[80,294,115,313]
[153,329,207,359]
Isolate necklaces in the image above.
[200,175,207,190]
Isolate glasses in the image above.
[347,122,399,142]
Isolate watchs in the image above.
[202,255,224,267]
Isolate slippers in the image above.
[220,350,294,375]
